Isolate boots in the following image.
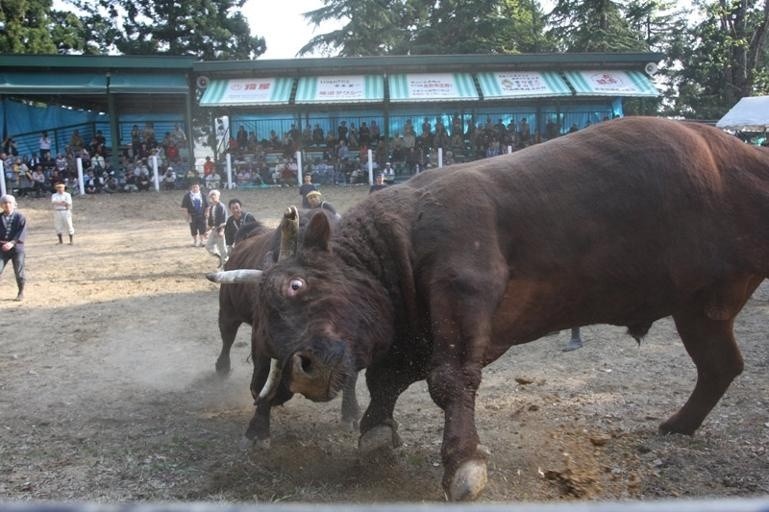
[14,283,25,301]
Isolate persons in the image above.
[563,325,583,352]
[52,184,74,245]
[306,189,341,222]
[205,189,228,269]
[180,182,209,247]
[224,199,256,258]
[300,174,315,211]
[0,194,26,302]
[0,113,620,199]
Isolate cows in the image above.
[202,114,769,503]
[209,200,360,443]
